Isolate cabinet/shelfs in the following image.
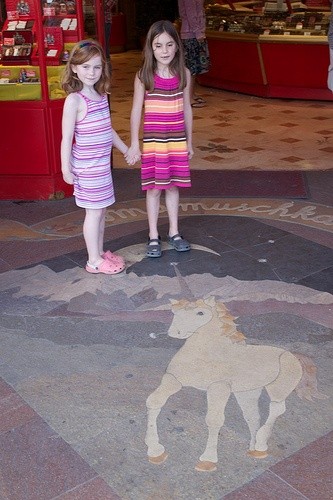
[0,13,79,102]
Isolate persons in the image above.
[92,0,115,59]
[124,20,193,257]
[61,39,141,275]
[178,0,209,107]
[327,8,333,92]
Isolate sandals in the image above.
[168,232,191,252]
[146,234,162,257]
[85,258,125,274]
[101,250,124,263]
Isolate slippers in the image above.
[191,97,208,108]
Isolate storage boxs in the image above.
[0,0,86,67]
